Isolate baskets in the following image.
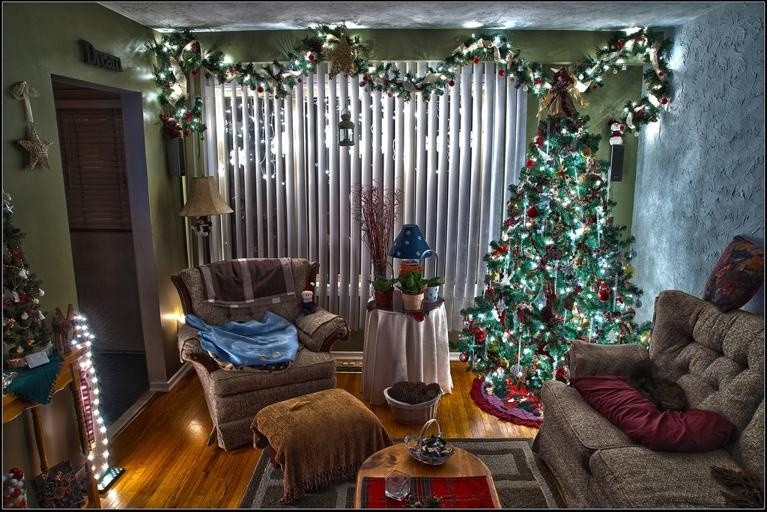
[384,387,443,425]
[405,418,455,466]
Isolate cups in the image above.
[385,470,410,501]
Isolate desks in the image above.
[3,343,101,509]
[360,285,454,405]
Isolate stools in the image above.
[252,390,393,505]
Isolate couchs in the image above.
[530,291,765,510]
[169,257,351,451]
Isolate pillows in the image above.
[702,235,764,314]
[569,376,740,453]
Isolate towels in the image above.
[194,257,297,309]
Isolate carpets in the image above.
[239,436,566,510]
[470,365,544,429]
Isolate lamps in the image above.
[178,175,234,264]
[388,225,433,275]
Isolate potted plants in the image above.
[367,269,446,311]
